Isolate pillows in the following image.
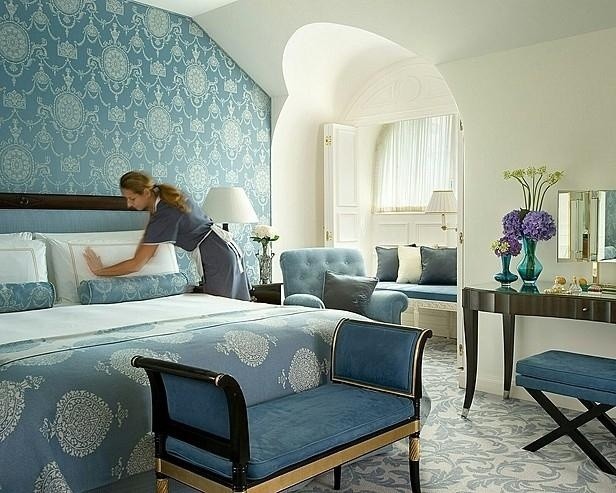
[322,270,379,315]
[376,243,457,285]
[0,229,203,312]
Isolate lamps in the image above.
[424,191,458,232]
[201,188,259,231]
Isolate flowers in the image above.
[502,167,567,278]
[250,225,279,285]
[492,238,523,257]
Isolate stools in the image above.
[515,350,616,474]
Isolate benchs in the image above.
[131,318,432,493]
[374,282,457,339]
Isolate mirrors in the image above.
[556,189,616,287]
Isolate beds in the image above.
[0,193,432,493]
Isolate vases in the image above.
[494,254,518,285]
[517,238,543,287]
[255,251,275,285]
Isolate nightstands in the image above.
[194,283,283,306]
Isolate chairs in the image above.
[280,248,408,325]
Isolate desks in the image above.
[461,282,616,419]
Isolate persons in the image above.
[83,171,252,301]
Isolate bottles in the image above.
[569,276,582,294]
